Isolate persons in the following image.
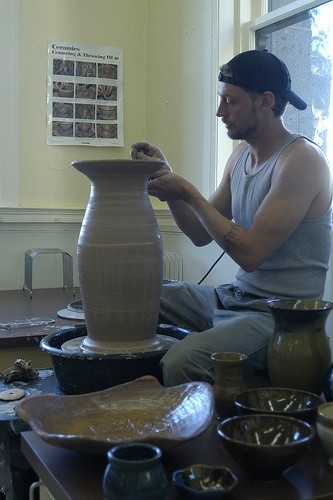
[130,49,333,389]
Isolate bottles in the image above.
[102,442,171,500]
[210,350,250,421]
[71,159,166,355]
[265,298,333,394]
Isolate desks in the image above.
[21,406,333,500]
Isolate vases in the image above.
[264,297,333,396]
[70,157,170,352]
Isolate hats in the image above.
[218,48,307,111]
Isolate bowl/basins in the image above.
[12,374,216,459]
[39,322,192,395]
[217,414,316,483]
[172,465,238,500]
[232,387,326,422]
[54,81,117,138]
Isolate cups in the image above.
[316,401,333,467]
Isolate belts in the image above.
[230,286,261,299]
